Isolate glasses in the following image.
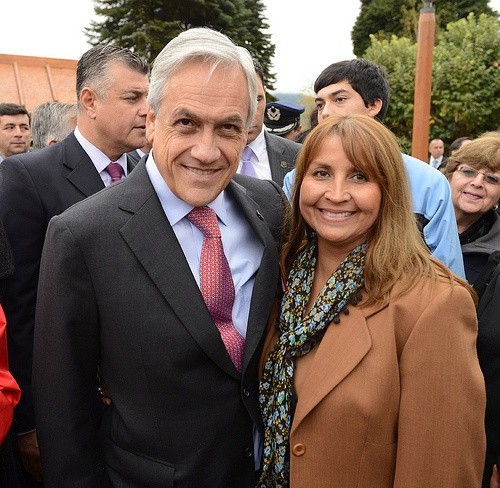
[455,165,500,185]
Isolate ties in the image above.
[432,161,438,168]
[239,146,258,178]
[187,207,245,374]
[104,163,123,186]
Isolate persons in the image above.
[0,45,500,488]
[96,114,487,488]
[31,28,291,488]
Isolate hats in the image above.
[263,101,305,135]
